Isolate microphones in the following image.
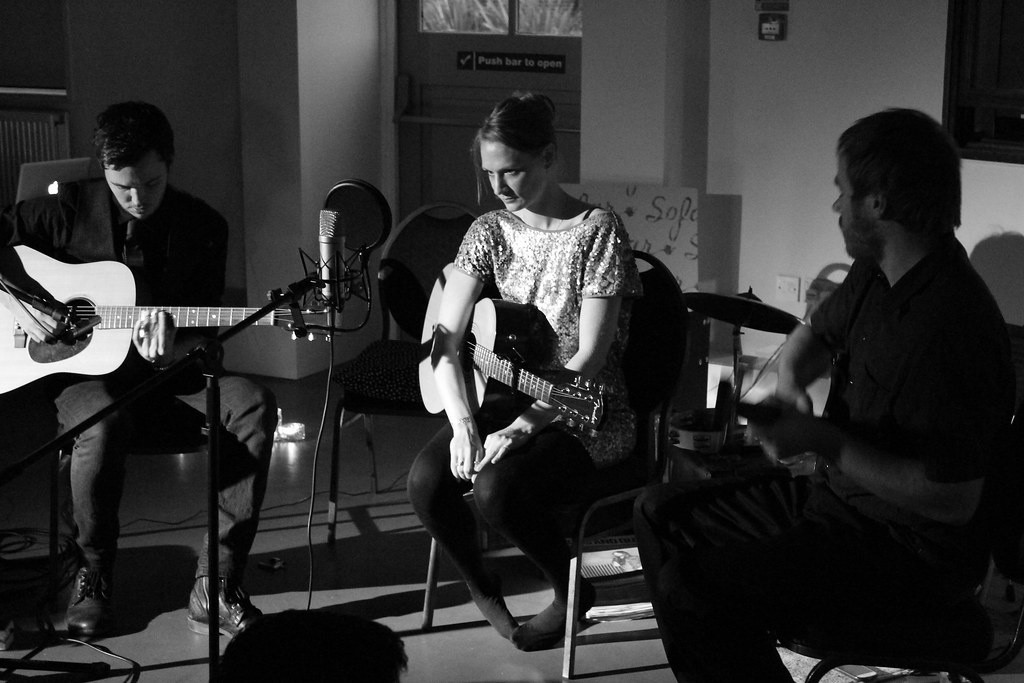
[320,210,348,334]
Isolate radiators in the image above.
[0,108,72,213]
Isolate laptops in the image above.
[16,157,92,205]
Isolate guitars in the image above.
[419,262,607,439]
[0,244,343,396]
[713,379,818,478]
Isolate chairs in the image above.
[421,249,689,679]
[327,202,477,552]
[767,323,1023,683]
[41,395,211,616]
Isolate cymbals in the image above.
[675,286,803,335]
[667,409,747,454]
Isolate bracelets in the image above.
[153,352,177,371]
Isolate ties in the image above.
[122,216,143,270]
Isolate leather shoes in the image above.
[63,559,114,637]
[187,577,263,638]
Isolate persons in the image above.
[634,108,1022,683]
[406,93,639,647]
[0,100,278,637]
